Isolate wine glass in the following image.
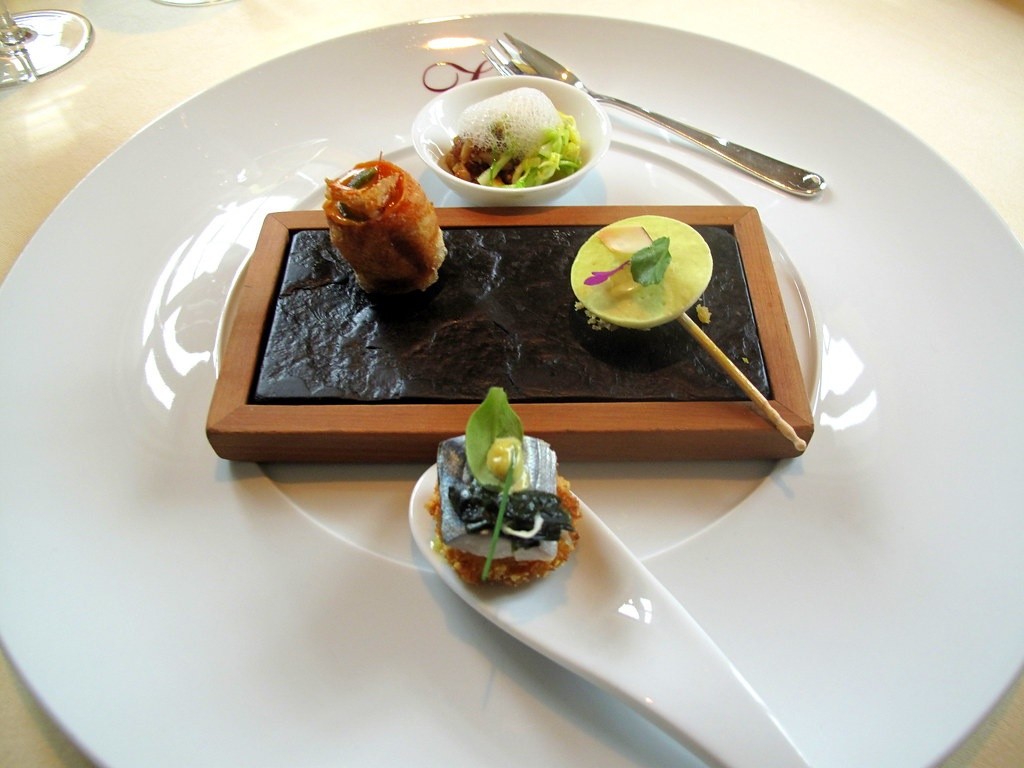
[0,0,94,83]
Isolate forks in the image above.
[481,31,826,198]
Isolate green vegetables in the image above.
[630,235,673,286]
[465,385,523,581]
[476,110,585,190]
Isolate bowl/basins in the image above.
[411,75,614,209]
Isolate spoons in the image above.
[407,463,808,768]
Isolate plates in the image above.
[0,10,1024,768]
